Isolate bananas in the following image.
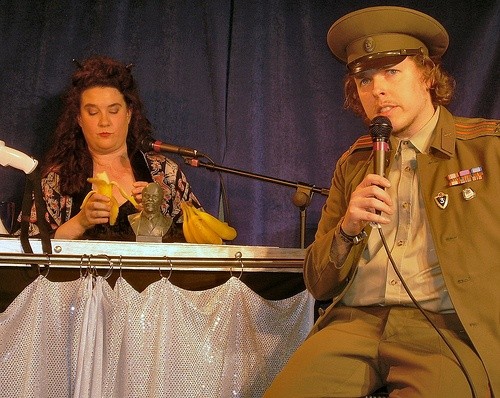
[80,172,129,226]
[181,200,237,245]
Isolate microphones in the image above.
[369,116,393,216]
[141,136,206,158]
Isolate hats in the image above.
[326,5,449,78]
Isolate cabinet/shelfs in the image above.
[0,236,319,398]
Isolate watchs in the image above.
[335,216,368,245]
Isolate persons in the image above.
[16,56,210,246]
[258,6,500,397]
[127,182,174,242]
